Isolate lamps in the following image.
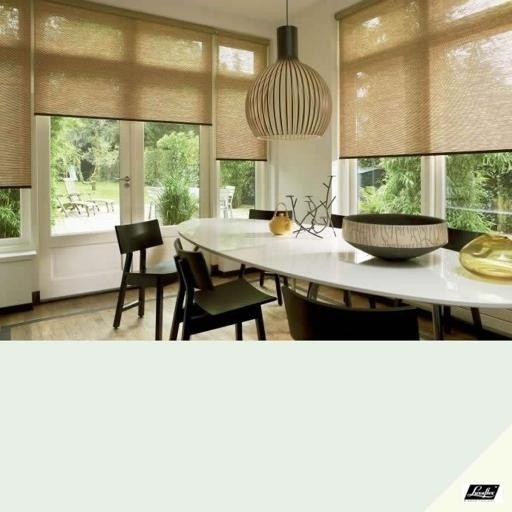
[245,0,333,142]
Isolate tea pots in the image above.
[266,202,293,237]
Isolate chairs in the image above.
[53,177,115,217]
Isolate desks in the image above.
[175,215,511,341]
